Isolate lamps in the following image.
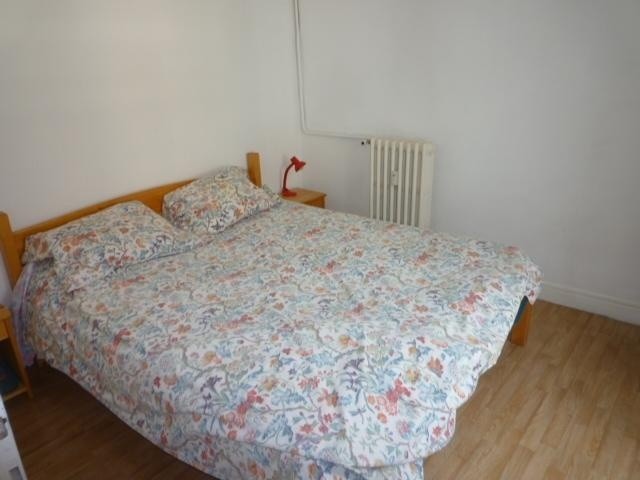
[279,155,306,198]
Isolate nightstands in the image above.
[278,188,327,209]
[0,306,34,405]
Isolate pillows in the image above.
[20,166,280,294]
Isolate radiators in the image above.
[368,138,434,232]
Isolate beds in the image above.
[0,152,540,480]
